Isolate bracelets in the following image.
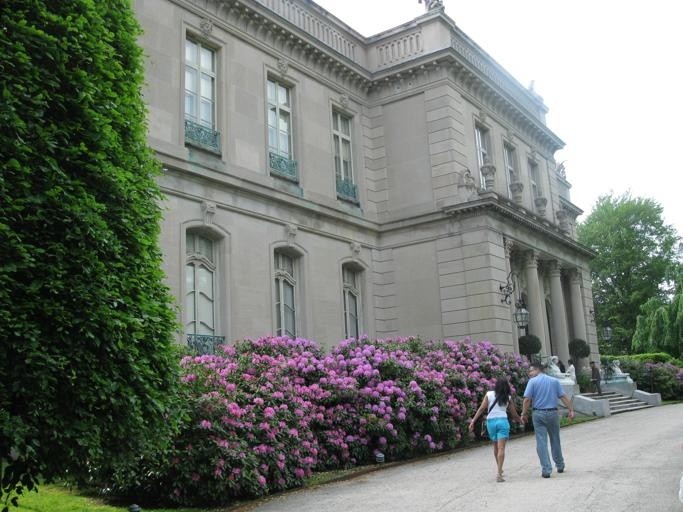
[520,412,527,416]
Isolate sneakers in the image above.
[496,471,504,481]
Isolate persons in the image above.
[566,359,577,385]
[589,361,602,396]
[468,378,522,483]
[520,362,574,478]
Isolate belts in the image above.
[533,408,558,411]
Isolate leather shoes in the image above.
[557,465,564,472]
[542,473,550,478]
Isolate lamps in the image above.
[590,311,612,341]
[501,269,531,329]
